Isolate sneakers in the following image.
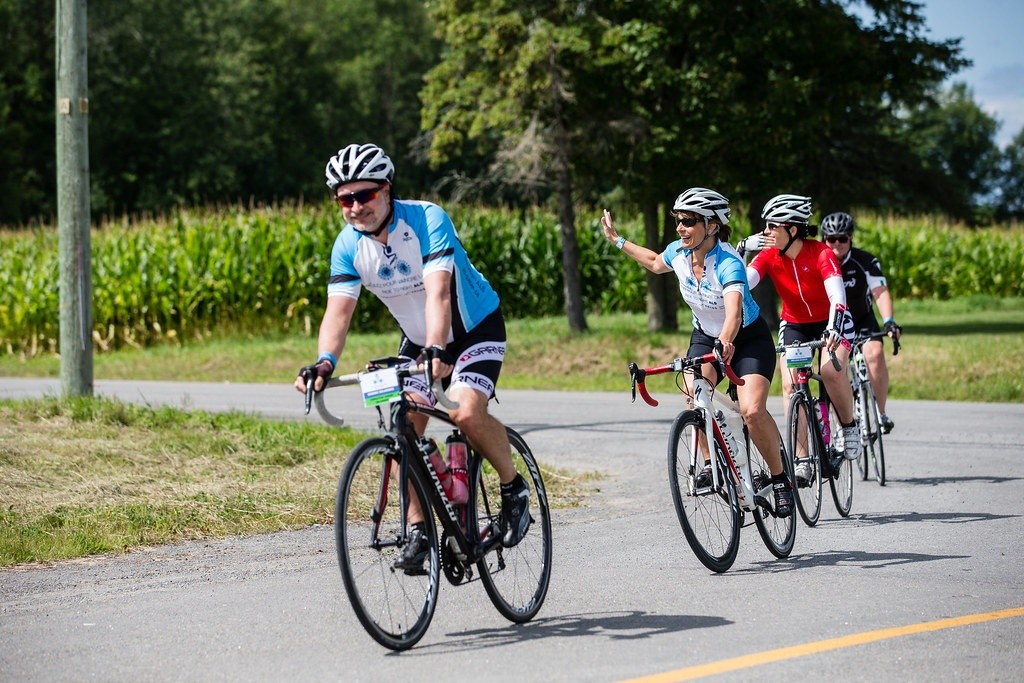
[771,481,794,518]
[796,462,812,483]
[694,466,724,488]
[842,421,861,460]
[833,427,845,453]
[501,473,530,548]
[881,416,894,431]
[395,527,433,569]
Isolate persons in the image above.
[818,211,901,454]
[735,194,863,483]
[601,188,796,520]
[295,144,530,567]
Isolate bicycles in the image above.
[775,331,853,528]
[854,327,901,486]
[631,339,798,574]
[304,349,552,650]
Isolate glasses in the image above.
[765,221,792,231]
[334,183,386,207]
[674,217,705,228]
[825,235,850,244]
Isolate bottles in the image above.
[420,436,454,494]
[715,410,738,457]
[817,397,830,444]
[446,428,468,504]
[812,396,824,429]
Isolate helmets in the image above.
[325,144,396,191]
[821,213,854,236]
[674,187,732,225]
[761,194,813,226]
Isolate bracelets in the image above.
[883,317,893,323]
[317,351,336,369]
[616,236,627,250]
